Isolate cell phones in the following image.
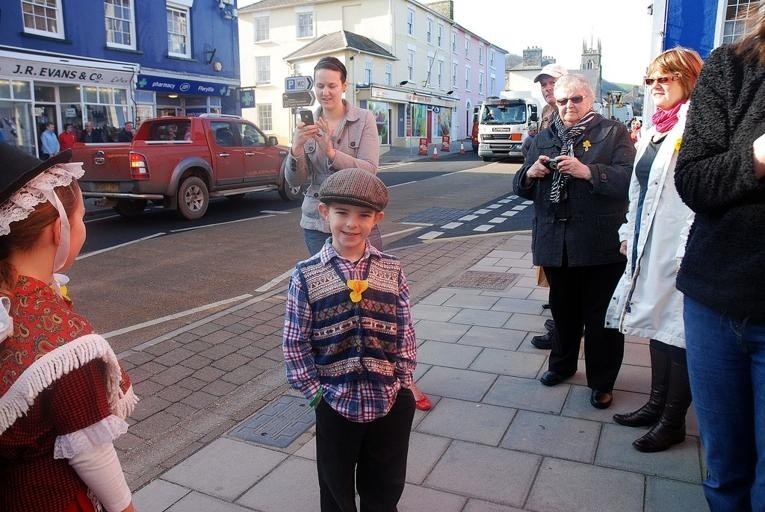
[300,110,314,126]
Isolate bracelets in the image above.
[309,384,323,408]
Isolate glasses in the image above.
[557,95,583,106]
[646,77,668,85]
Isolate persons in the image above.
[0,116,187,160]
[676,0,765,510]
[282,56,433,410]
[281,168,417,512]
[0,142,140,512]
[511,46,703,451]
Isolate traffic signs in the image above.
[281,91,314,108]
[282,77,317,93]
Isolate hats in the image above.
[534,64,565,82]
[319,168,389,211]
[0,143,72,202]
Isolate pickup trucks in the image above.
[67,111,306,222]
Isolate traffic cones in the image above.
[430,143,440,160]
[459,138,466,157]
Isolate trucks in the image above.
[471,106,479,151]
[477,86,544,161]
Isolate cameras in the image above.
[543,159,562,171]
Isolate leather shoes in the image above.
[591,388,613,408]
[531,319,576,386]
[416,392,431,410]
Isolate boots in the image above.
[613,346,692,452]
